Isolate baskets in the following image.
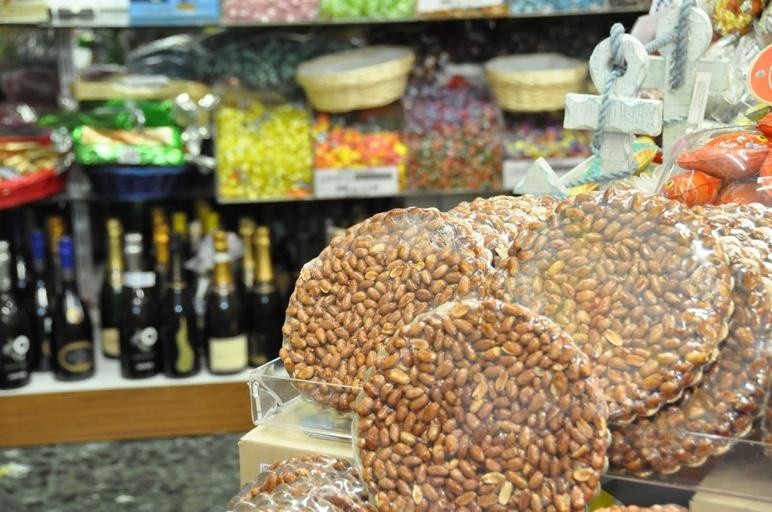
[294,44,416,114]
[485,52,589,113]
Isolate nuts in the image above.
[223,186,772,511]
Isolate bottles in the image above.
[203,230,247,375]
[158,232,200,377]
[97,203,284,379]
[121,232,160,378]
[0,216,95,390]
[0,239,32,387]
[50,234,93,381]
[249,226,281,366]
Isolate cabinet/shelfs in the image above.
[0,1,660,448]
